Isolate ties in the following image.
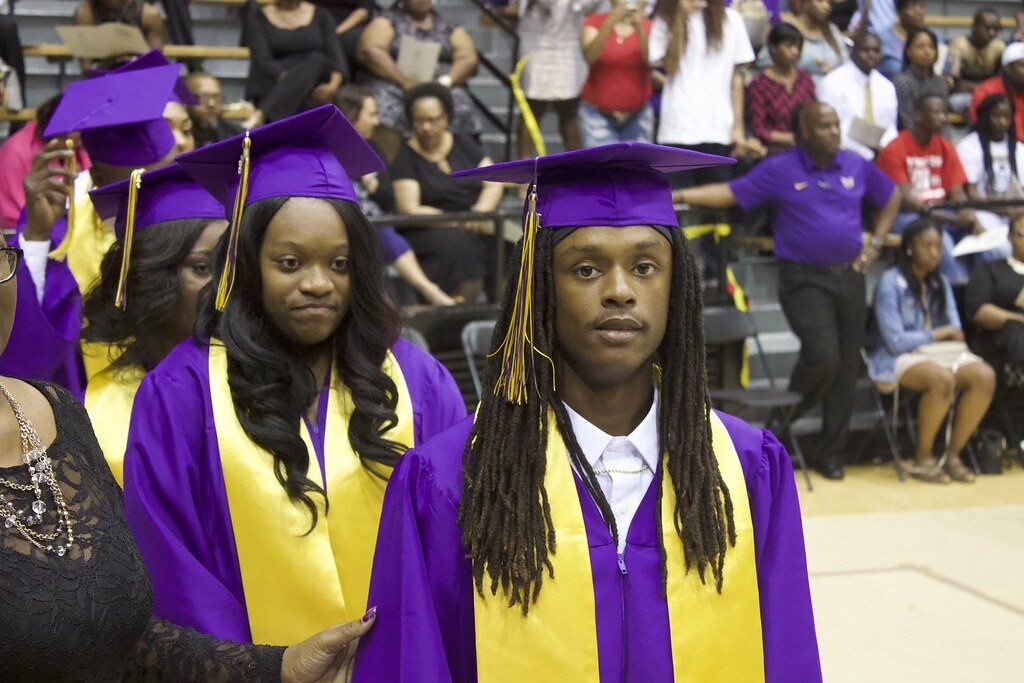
[864,78,875,127]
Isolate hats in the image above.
[1001,42,1024,65]
[452,143,738,406]
[86,145,230,313]
[176,104,387,310]
[43,49,202,262]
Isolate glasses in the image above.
[0,248,24,284]
[409,115,448,124]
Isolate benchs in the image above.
[0,0,1024,169]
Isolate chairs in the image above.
[461,319,498,403]
[856,280,983,481]
[702,305,814,493]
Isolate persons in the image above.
[0,0,247,490]
[242,0,525,318]
[121,102,469,646]
[1,230,379,683]
[517,1,1024,485]
[349,141,824,682]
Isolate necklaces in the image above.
[594,465,649,475]
[0,386,74,557]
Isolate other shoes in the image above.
[230,123,255,133]
[915,452,951,484]
[804,444,844,480]
[755,235,778,256]
[767,412,789,446]
[701,289,751,306]
[948,458,975,483]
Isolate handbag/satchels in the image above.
[966,428,1005,475]
